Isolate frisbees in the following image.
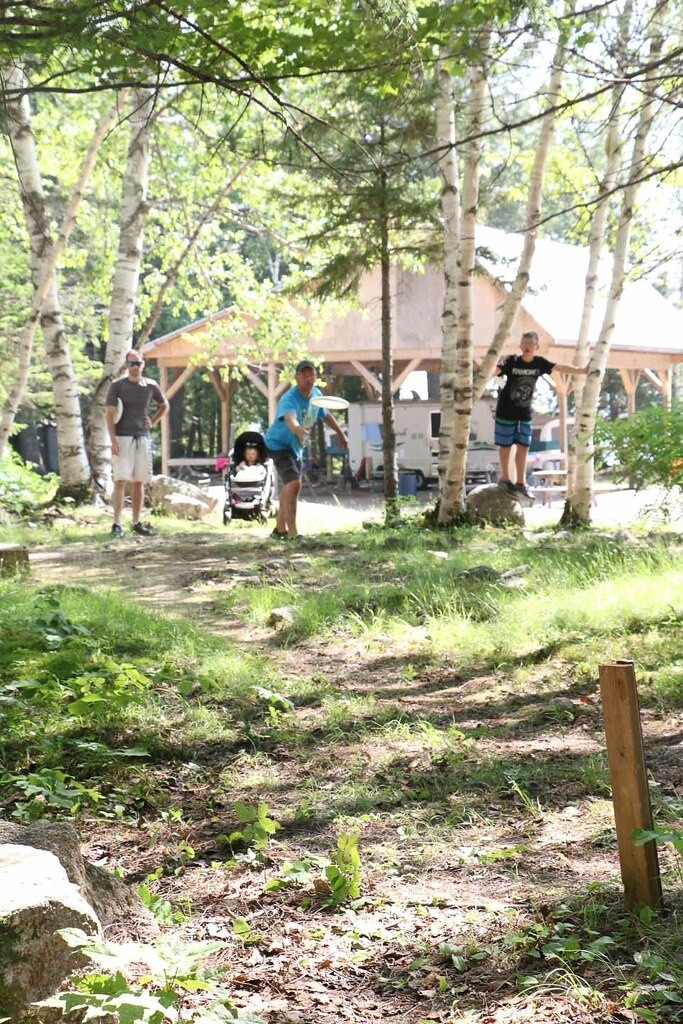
[310,396,349,410]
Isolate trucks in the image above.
[347,399,504,491]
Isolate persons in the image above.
[472,331,589,500]
[262,361,349,542]
[234,447,265,480]
[105,349,171,537]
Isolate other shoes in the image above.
[133,521,150,534]
[289,535,309,545]
[269,528,288,541]
[110,524,125,538]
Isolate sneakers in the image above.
[498,479,521,500]
[516,481,536,500]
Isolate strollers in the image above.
[223,426,280,523]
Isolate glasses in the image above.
[127,360,143,367]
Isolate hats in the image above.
[296,360,315,375]
